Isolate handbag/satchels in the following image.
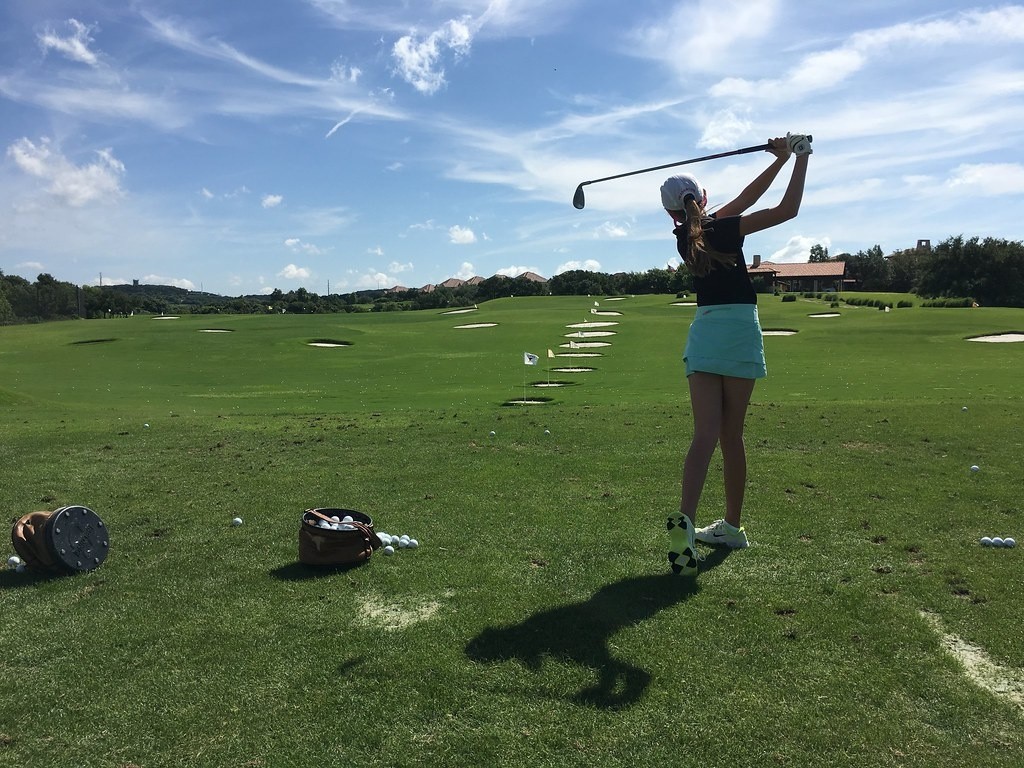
[11,506,110,575]
[298,506,383,564]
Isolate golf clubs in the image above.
[573,134,813,210]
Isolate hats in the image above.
[660,172,704,211]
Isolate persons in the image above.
[660,132,813,588]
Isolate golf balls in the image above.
[992,537,1003,548]
[490,430,497,436]
[1004,538,1016,548]
[970,465,980,473]
[7,554,21,566]
[314,515,360,529]
[410,539,419,548]
[392,535,399,544]
[401,534,410,541]
[385,545,395,556]
[143,423,150,429]
[544,429,551,436]
[232,516,244,527]
[961,405,968,413]
[376,532,392,545]
[399,540,409,549]
[980,536,991,548]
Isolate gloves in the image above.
[786,131,814,158]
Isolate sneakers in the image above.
[666,511,699,579]
[694,519,749,549]
